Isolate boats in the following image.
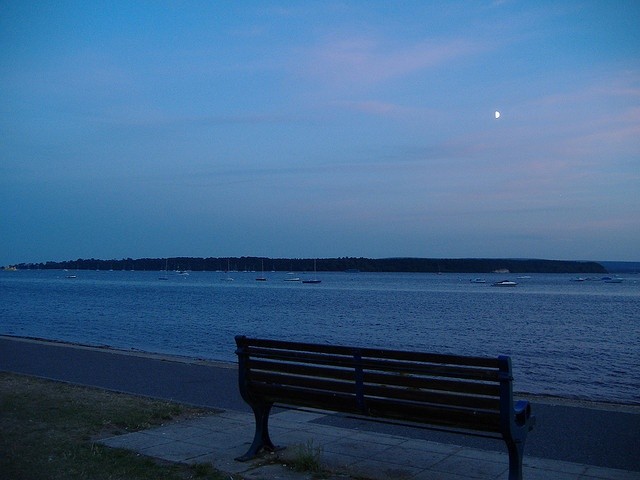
[284,278,300,281]
[255,277,267,280]
[471,278,486,283]
[492,280,518,286]
[605,274,624,283]
[575,277,584,281]
[303,280,321,283]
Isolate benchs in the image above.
[235,335,537,480]
